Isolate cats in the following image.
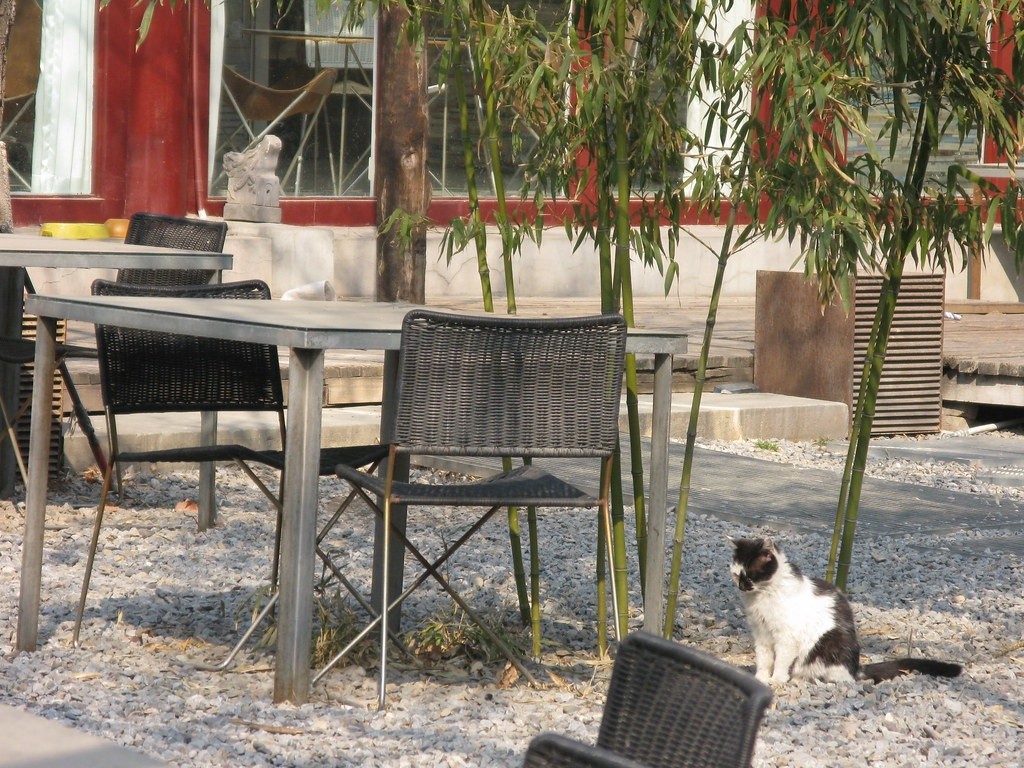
[724,534,964,687]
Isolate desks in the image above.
[0,235,234,534]
[16,293,686,706]
[241,28,501,196]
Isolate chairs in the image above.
[312,309,630,716]
[71,280,428,669]
[209,62,338,196]
[0,218,114,502]
[593,629,774,768]
[114,212,229,287]
[521,732,652,768]
